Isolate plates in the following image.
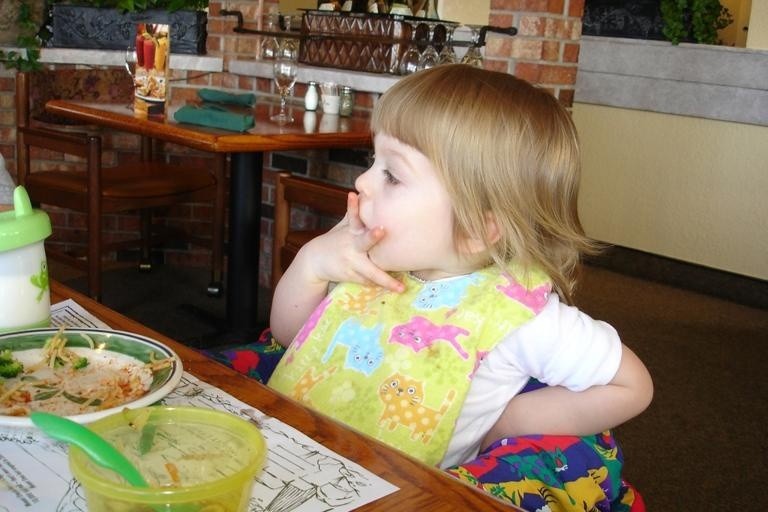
[0,326,185,428]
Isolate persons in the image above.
[265,60,657,473]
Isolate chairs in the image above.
[16,65,226,304]
[270,171,360,304]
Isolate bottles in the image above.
[0,185,52,331]
[304,81,318,110]
[338,85,355,116]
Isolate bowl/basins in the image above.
[67,407,268,511]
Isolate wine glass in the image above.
[402,20,487,75]
[256,10,300,62]
[271,56,297,124]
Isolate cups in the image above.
[320,94,340,114]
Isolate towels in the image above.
[174,105,256,132]
[197,87,257,107]
